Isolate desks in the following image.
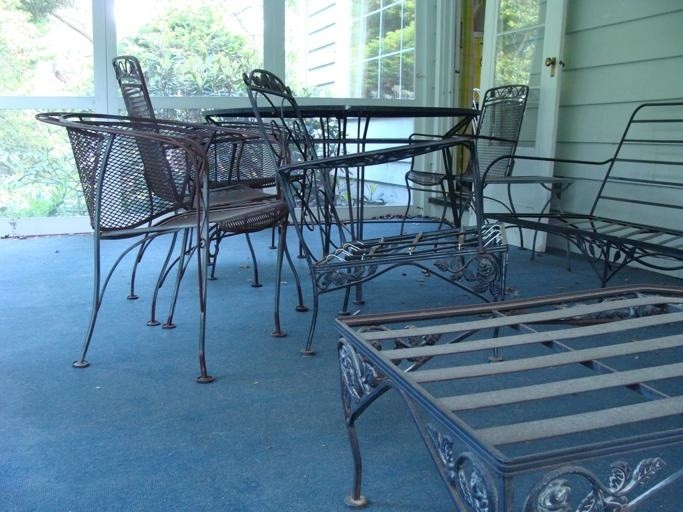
[200,104,480,306]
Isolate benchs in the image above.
[482,101,683,319]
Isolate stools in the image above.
[335,283,683,512]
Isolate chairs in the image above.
[400,83,529,251]
[35,112,290,383]
[242,68,510,361]
[112,55,311,324]
